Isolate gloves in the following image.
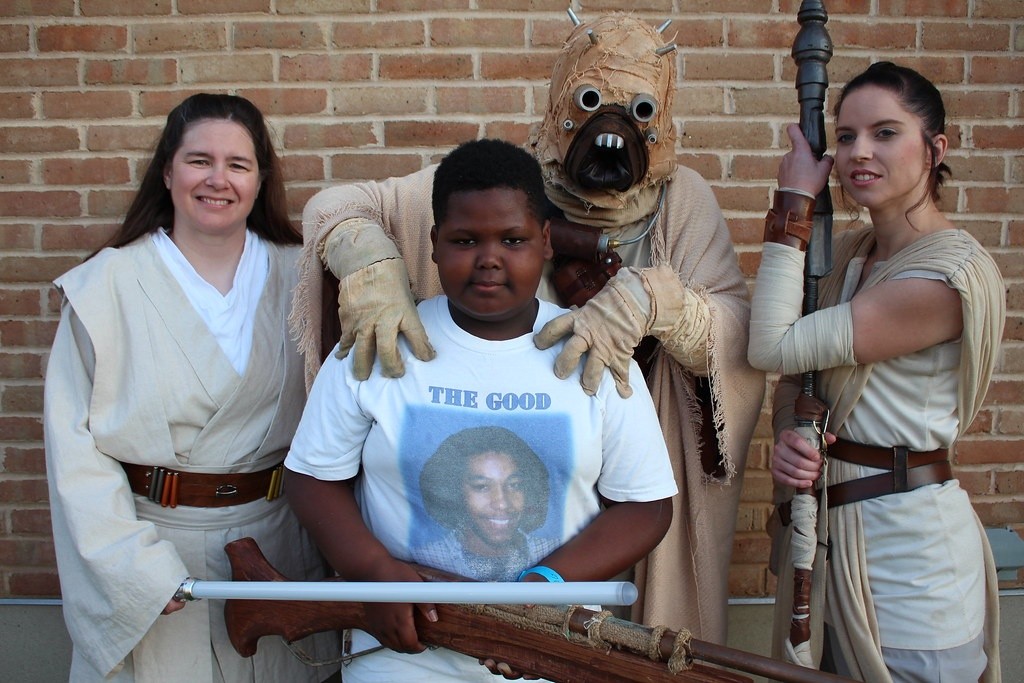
[532,266,651,398]
[325,219,437,381]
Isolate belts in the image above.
[777,436,952,527]
[117,461,288,508]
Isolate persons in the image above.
[283,136,678,683]
[413,425,563,583]
[747,61,1005,683]
[43,92,343,683]
[288,6,767,672]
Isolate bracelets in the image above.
[517,566,572,608]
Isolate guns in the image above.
[219,529,865,683]
